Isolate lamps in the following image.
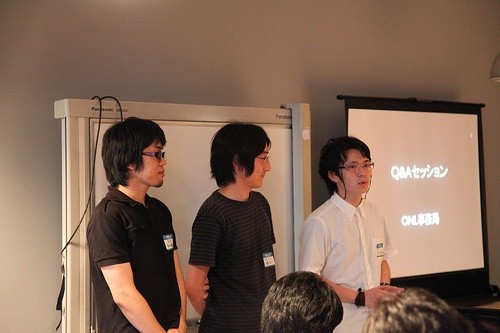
[489,52,500,81]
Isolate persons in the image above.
[362,288,473,333]
[261,272,343,333]
[299,137,404,333]
[185,122,277,333]
[87,117,187,333]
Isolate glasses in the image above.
[337,162,375,172]
[257,155,269,163]
[142,150,166,160]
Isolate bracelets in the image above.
[380,282,389,285]
[355,288,365,306]
